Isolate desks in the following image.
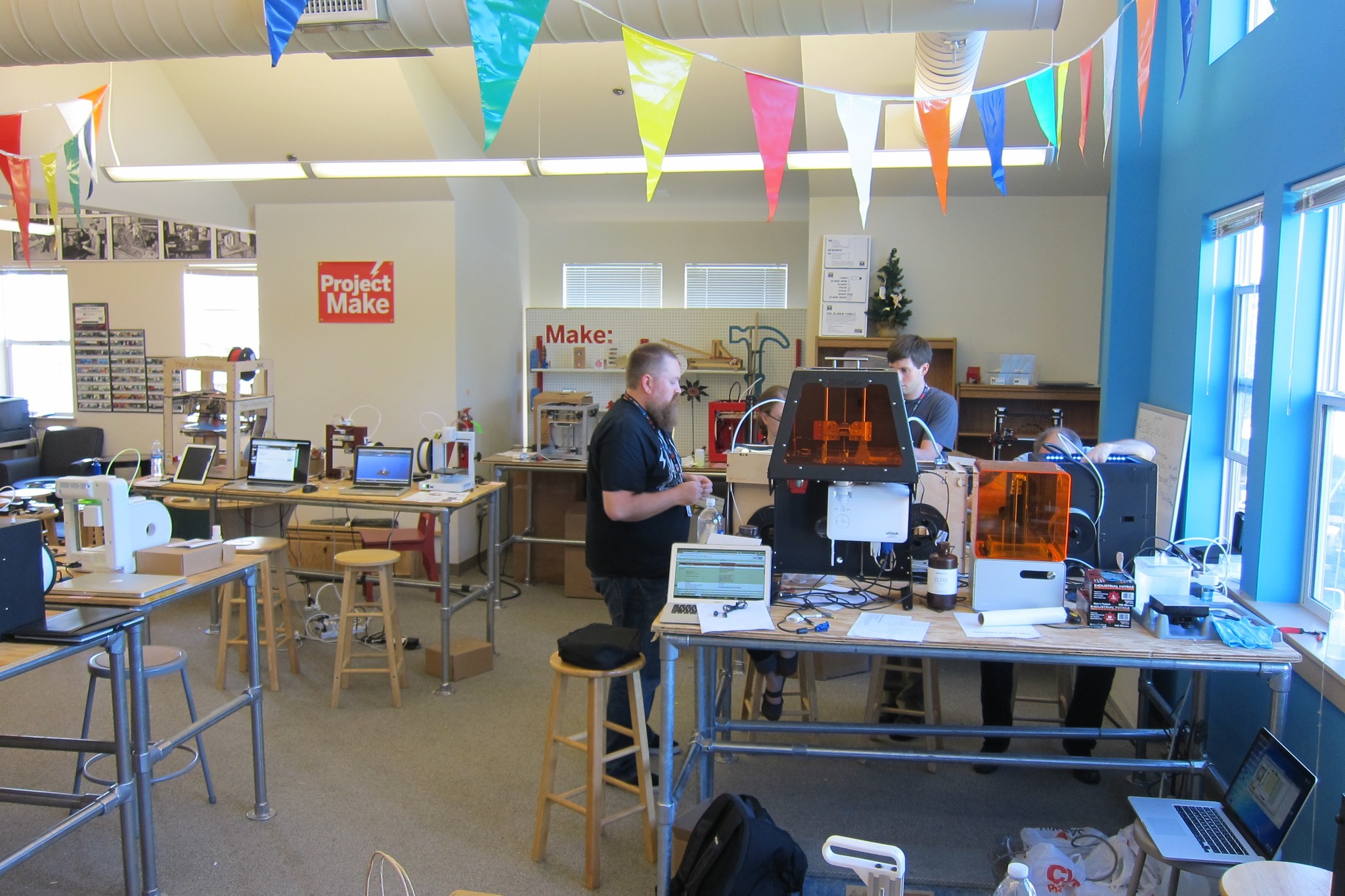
[478,449,732,589]
[93,454,152,481]
[0,610,142,896]
[651,571,1303,896]
[125,469,511,696]
[0,544,278,826]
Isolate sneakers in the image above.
[648,733,682,755]
[627,771,660,794]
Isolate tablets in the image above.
[172,443,216,485]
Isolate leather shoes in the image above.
[1062,738,1102,785]
[973,746,999,774]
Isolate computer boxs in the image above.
[0,517,47,634]
[1027,452,1158,578]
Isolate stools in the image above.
[329,548,409,709]
[529,648,657,892]
[217,535,301,692]
[852,650,947,773]
[70,645,217,815]
[1218,858,1333,896]
[1125,815,1282,895]
[736,646,817,757]
[1006,662,1075,753]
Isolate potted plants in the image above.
[865,247,913,338]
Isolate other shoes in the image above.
[889,714,926,741]
[761,675,786,721]
[879,714,898,724]
[775,651,799,676]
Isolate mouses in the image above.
[302,485,318,493]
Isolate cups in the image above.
[695,445,706,470]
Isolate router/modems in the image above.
[826,481,910,566]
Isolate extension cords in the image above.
[320,626,364,639]
[304,605,321,611]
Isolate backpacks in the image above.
[654,795,809,896]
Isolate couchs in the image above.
[0,424,104,493]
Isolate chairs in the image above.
[358,510,443,604]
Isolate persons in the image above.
[81,224,102,261]
[724,385,802,720]
[39,235,53,254]
[128,222,143,247]
[875,333,959,741]
[971,427,1158,784]
[222,230,236,251]
[147,232,159,256]
[587,343,715,792]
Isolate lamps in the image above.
[0,197,60,236]
[103,30,1056,186]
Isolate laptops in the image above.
[1127,726,1318,865]
[339,445,415,497]
[221,437,311,494]
[659,542,772,624]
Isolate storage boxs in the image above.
[563,498,605,600]
[510,468,583,586]
[1076,587,1132,628]
[533,391,593,445]
[424,637,494,682]
[1134,556,1195,612]
[1084,568,1136,607]
[133,538,237,578]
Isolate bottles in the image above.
[151,441,164,477]
[926,542,958,614]
[993,862,1036,895]
[698,498,724,545]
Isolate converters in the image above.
[404,638,418,650]
[900,585,913,611]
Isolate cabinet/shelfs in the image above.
[955,381,1103,464]
[815,335,957,400]
